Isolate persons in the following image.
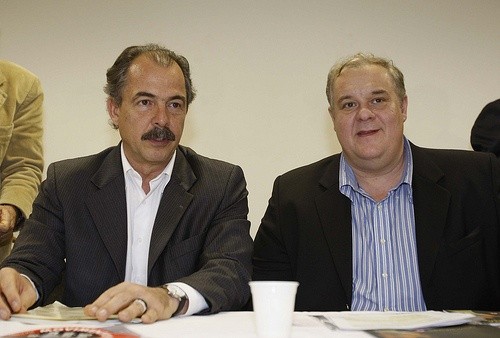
[0,59,44,263]
[252,52,500,312]
[0,43,253,324]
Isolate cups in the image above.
[249,281,300,338]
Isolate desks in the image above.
[0,310,500,338]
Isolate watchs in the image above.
[158,284,186,317]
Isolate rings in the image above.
[135,298,148,312]
